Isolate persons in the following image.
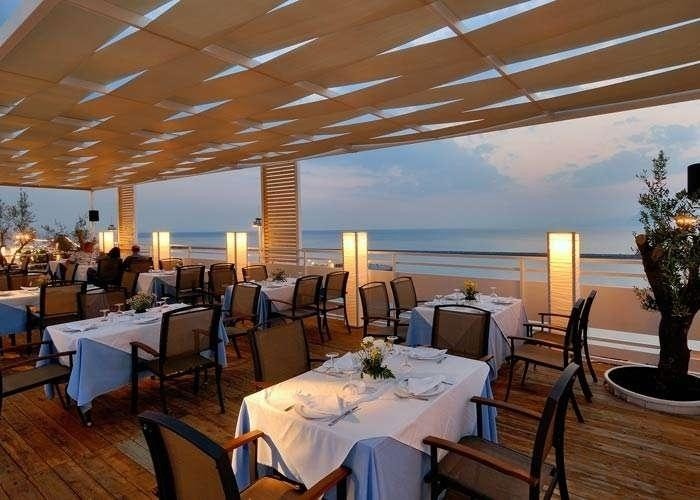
[65,242,149,288]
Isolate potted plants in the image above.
[604,148,700,416]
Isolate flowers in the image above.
[461,279,480,299]
[356,336,393,379]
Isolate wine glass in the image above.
[99,309,109,330]
[113,303,125,322]
[253,279,295,288]
[432,287,500,306]
[386,335,398,356]
[160,297,171,312]
[156,300,165,318]
[340,370,358,405]
[398,343,413,370]
[325,352,339,382]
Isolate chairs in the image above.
[137,411,349,499]
[421,365,583,500]
[359,276,428,344]
[414,304,497,381]
[501,290,597,423]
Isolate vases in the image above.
[363,372,384,381]
[466,300,479,305]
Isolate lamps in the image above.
[226,232,248,282]
[547,230,583,334]
[152,231,170,271]
[99,230,118,254]
[343,231,368,327]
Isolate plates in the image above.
[393,373,445,398]
[128,312,162,324]
[408,346,441,360]
[295,393,345,420]
